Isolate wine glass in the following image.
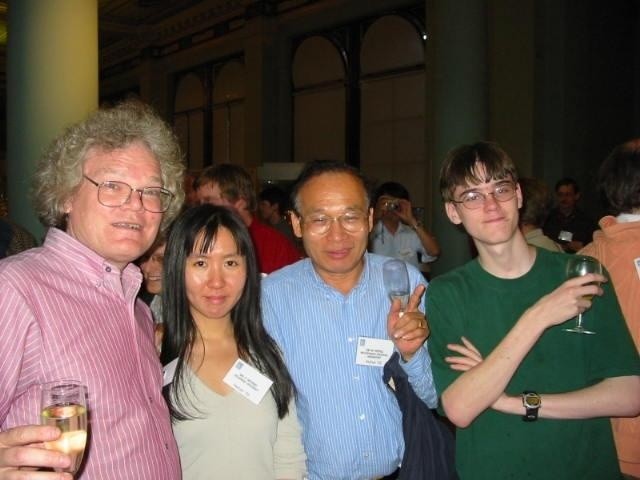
[563,257,603,337]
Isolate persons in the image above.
[139,137,638,479]
[0,101,190,480]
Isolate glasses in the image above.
[305,210,366,234]
[82,174,174,213]
[450,183,517,209]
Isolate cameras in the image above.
[386,201,401,212]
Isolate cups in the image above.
[382,260,412,313]
[40,381,88,476]
